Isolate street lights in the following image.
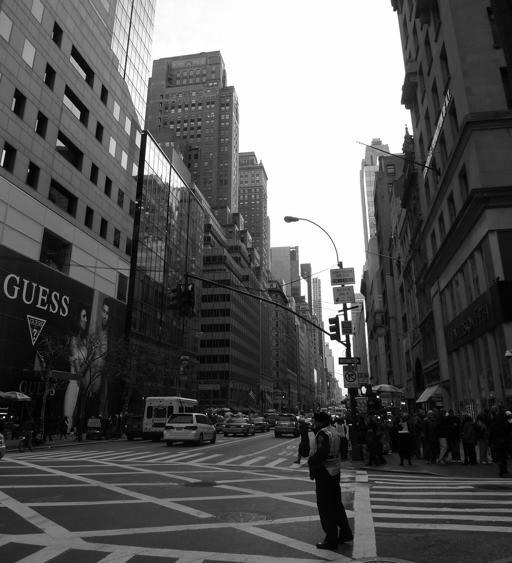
[280,213,365,461]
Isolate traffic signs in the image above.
[338,357,360,365]
[341,320,352,329]
[342,328,353,335]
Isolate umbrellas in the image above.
[372,383,405,392]
[4,391,32,407]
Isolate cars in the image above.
[0,432,7,460]
[213,406,349,437]
[162,409,218,448]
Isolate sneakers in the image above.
[335,533,354,543]
[316,540,339,550]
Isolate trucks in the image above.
[122,385,161,442]
[140,395,201,442]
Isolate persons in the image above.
[63,308,96,434]
[308,411,354,549]
[0,408,167,450]
[83,298,116,429]
[292,401,512,477]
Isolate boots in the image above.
[294,458,301,464]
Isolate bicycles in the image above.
[17,430,34,452]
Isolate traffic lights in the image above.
[186,282,196,318]
[360,382,373,397]
[328,315,340,342]
[178,282,187,317]
